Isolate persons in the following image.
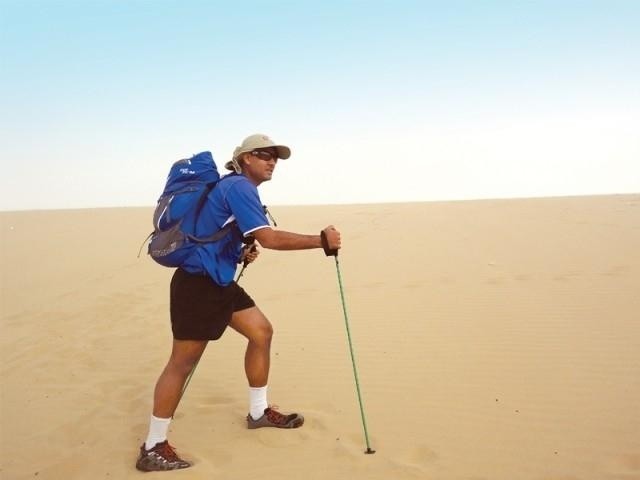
[134,132,343,473]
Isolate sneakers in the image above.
[245,403,305,430]
[135,438,191,473]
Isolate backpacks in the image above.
[146,150,249,268]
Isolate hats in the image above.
[232,133,292,161]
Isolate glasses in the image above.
[246,149,279,164]
